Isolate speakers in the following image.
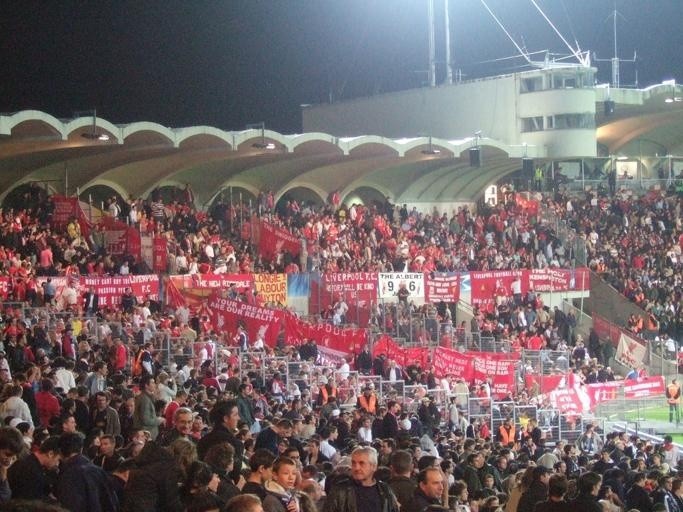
[469,149,480,167]
[604,101,615,116]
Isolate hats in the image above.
[532,466,553,478]
